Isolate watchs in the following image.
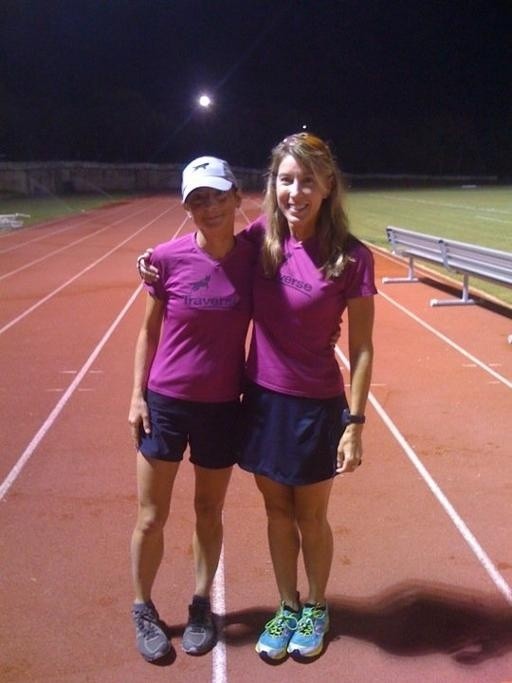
[340,408,368,427]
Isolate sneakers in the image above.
[287,598,331,660]
[254,593,300,664]
[131,599,172,664]
[178,593,217,656]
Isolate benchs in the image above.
[381,224,512,344]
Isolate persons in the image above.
[134,129,381,664]
[121,150,344,664]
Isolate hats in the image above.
[180,155,239,207]
